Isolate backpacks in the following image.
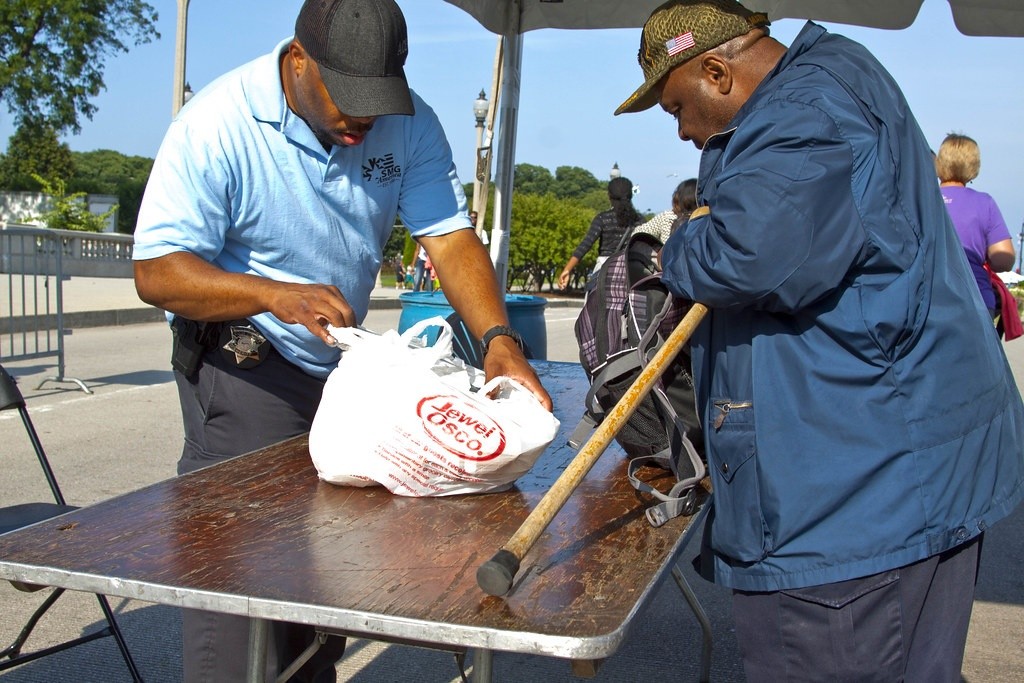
[574,230,708,525]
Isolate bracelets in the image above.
[478,323,525,365]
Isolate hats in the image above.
[614,1,771,115]
[295,1,416,118]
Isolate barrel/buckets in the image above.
[398,286,547,364]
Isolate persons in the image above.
[934,133,1024,342]
[557,176,699,292]
[130,0,554,478]
[574,0,1024,683]
[395,239,439,294]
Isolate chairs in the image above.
[0,364,145,683]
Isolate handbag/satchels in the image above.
[307,317,562,499]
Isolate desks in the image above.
[0,359,712,683]
